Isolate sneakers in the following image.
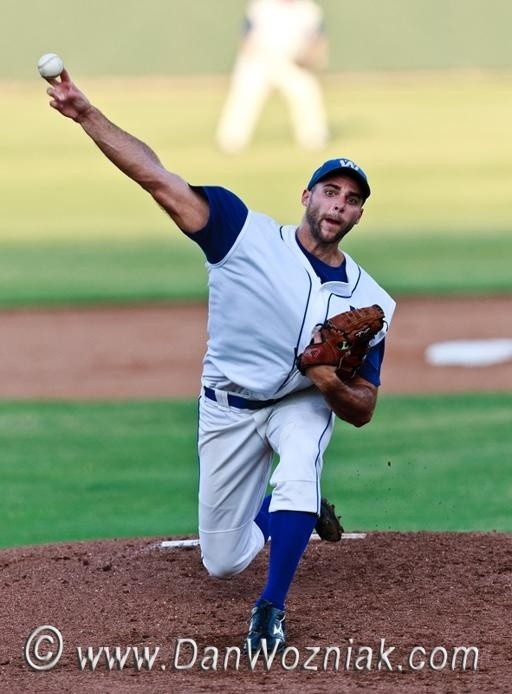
[242,608,287,653]
[314,497,345,544]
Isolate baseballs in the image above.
[38,53,64,79]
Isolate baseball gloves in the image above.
[294,304,389,384]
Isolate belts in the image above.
[204,388,283,410]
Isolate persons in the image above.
[40,64,398,656]
[213,0,333,152]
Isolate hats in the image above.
[307,156,371,207]
